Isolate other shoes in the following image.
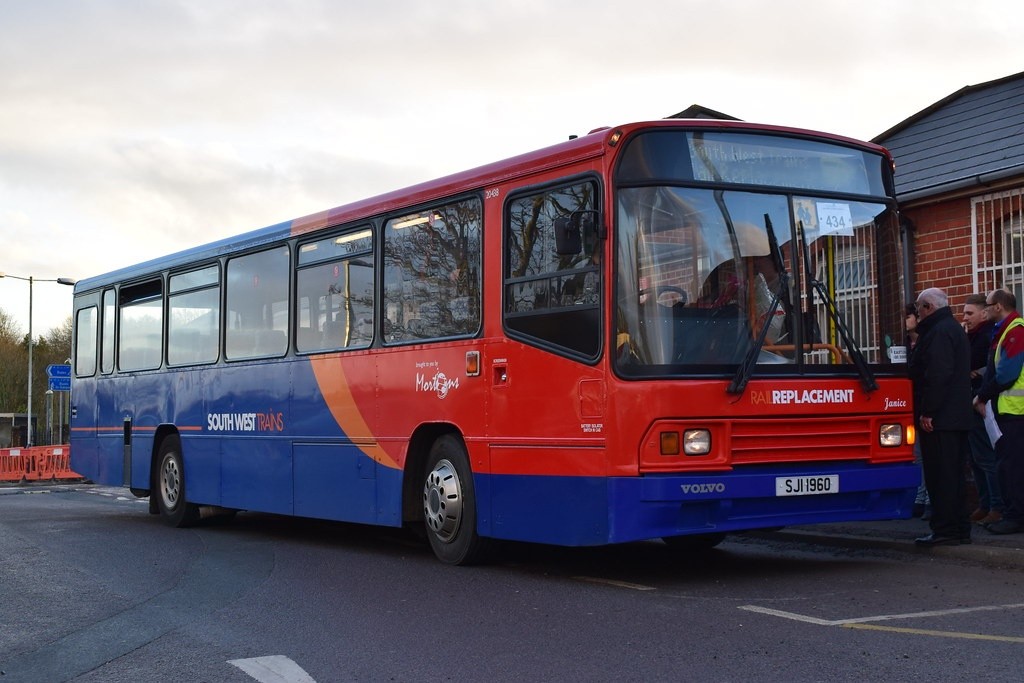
[911,503,932,520]
[965,507,1003,525]
[987,520,1024,534]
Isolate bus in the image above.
[65,117,924,569]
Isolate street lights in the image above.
[0,269,77,450]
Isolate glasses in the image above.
[913,303,929,308]
[984,303,997,311]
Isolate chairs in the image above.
[320,320,347,349]
[301,327,318,352]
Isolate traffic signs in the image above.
[45,364,71,392]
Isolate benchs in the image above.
[227,328,286,359]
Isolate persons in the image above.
[735,247,822,360]
[906,302,932,520]
[963,294,1004,526]
[552,241,599,301]
[909,287,972,547]
[973,288,1024,534]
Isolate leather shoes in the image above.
[915,533,970,546]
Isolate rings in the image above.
[926,424,928,426]
